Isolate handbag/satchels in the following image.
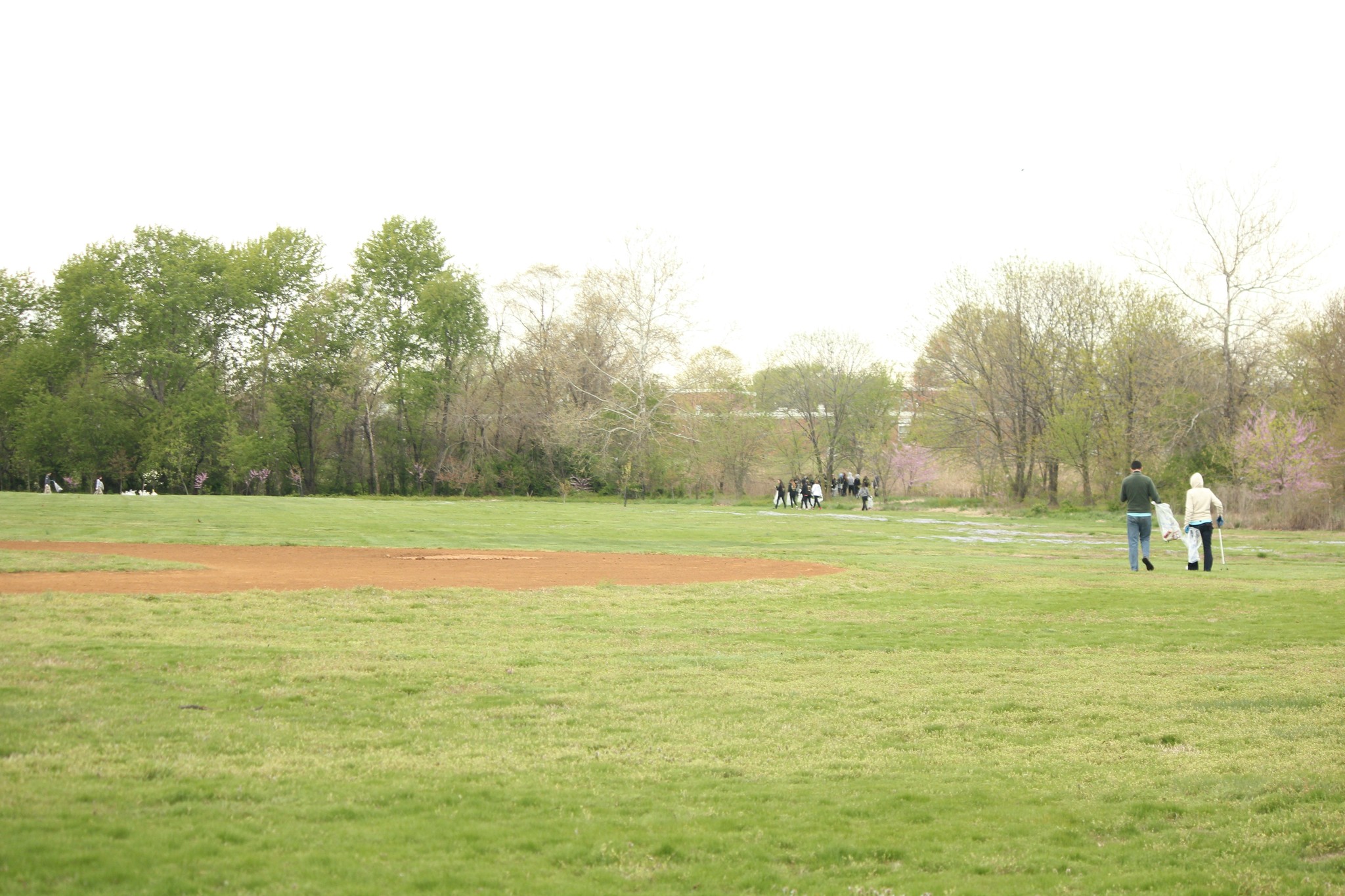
[797,489,804,504]
[810,496,823,507]
[867,496,874,510]
[773,490,784,507]
[1148,497,1182,543]
[1180,526,1202,564]
[801,497,812,510]
[786,492,795,506]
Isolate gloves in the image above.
[1183,521,1190,533]
[1216,515,1224,528]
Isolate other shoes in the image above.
[1141,556,1155,572]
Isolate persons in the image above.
[44,471,55,494]
[772,471,880,511]
[1120,461,1162,571]
[1183,472,1223,572]
[93,476,104,494]
